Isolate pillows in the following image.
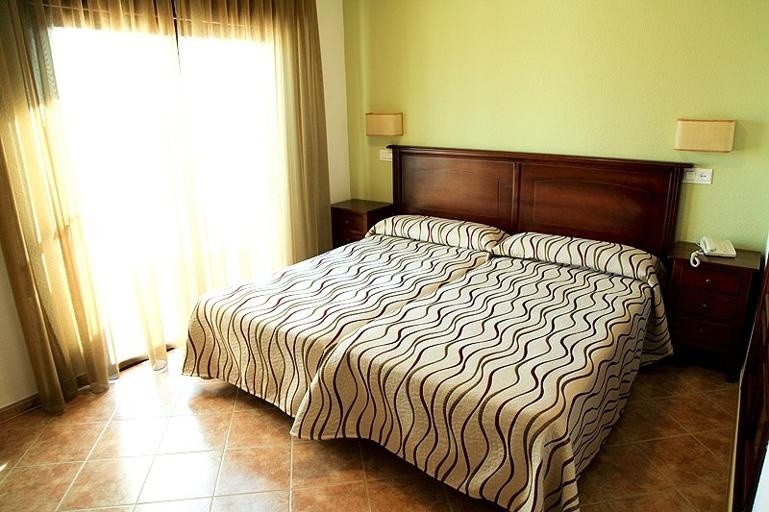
[493,232,656,280]
[365,214,511,252]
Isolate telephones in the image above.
[700,236,737,258]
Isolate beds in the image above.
[182,145,697,512]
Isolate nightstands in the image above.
[331,199,392,249]
[666,241,762,383]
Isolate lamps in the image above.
[365,112,403,137]
[674,118,736,152]
